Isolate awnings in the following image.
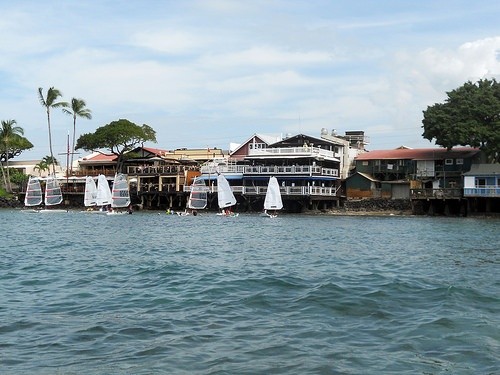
[194,175,242,181]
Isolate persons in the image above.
[87,206,134,214]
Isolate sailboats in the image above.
[95,174,113,214]
[20,175,43,212]
[81,176,103,215]
[105,170,131,216]
[257,176,284,218]
[40,173,69,214]
[173,174,207,216]
[215,174,240,218]
